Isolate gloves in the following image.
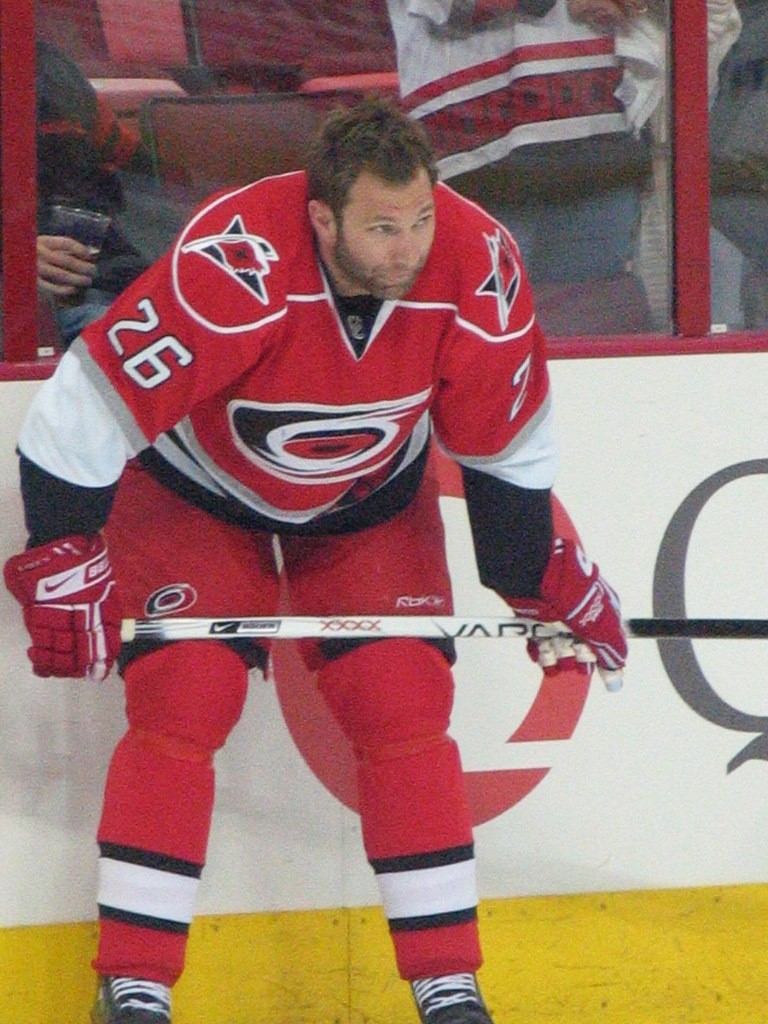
[3,534,122,683]
[500,536,627,695]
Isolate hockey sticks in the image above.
[114,618,768,639]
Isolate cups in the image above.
[46,206,111,308]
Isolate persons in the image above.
[0,1,768,360]
[4,104,630,1024]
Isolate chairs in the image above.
[35,0,402,204]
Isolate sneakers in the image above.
[90,976,171,1024]
[410,973,495,1024]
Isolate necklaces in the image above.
[318,255,377,341]
